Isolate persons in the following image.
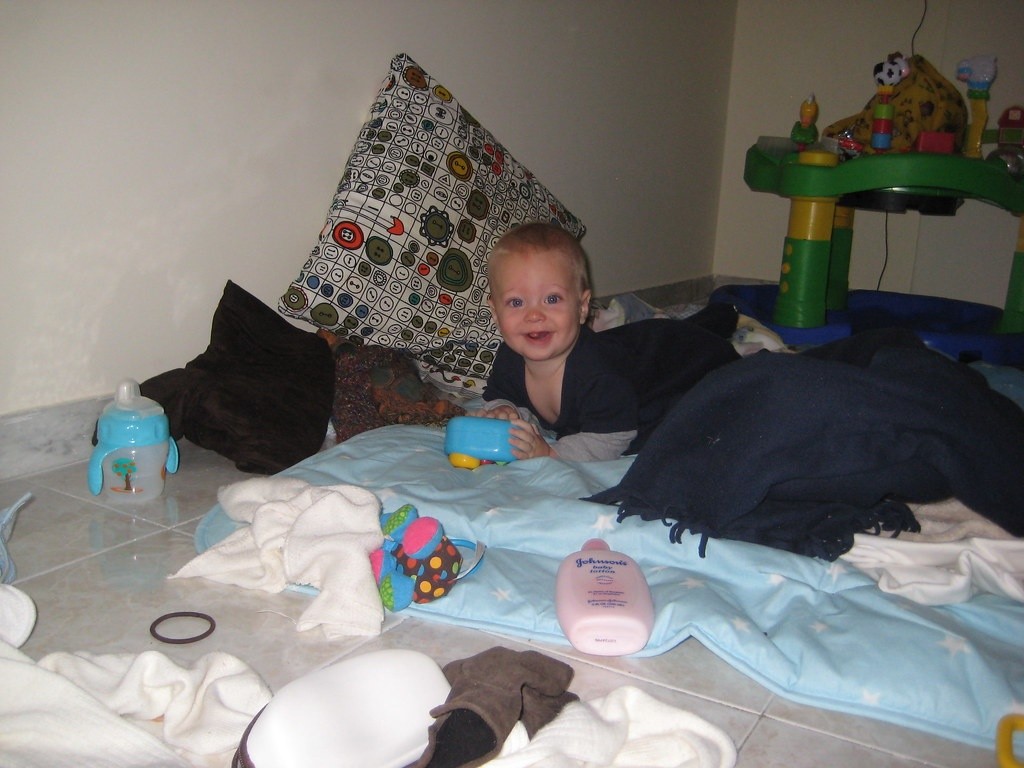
[469,222,743,466]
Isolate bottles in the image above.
[87,379,179,508]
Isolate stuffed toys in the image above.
[373,503,464,612]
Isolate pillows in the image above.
[91,279,335,477]
[313,329,468,444]
[273,54,589,406]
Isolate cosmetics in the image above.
[555,538,656,657]
